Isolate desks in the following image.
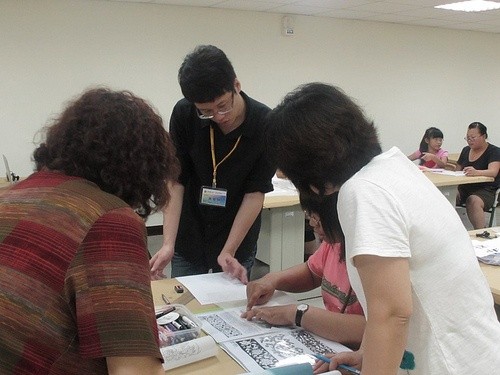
[255,165,494,272]
[468,225,500,305]
[151,272,362,375]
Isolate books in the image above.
[192,303,354,375]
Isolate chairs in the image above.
[456,189,500,227]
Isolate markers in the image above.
[161,294,170,304]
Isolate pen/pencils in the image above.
[310,352,361,374]
[156,306,196,340]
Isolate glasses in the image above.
[464,134,483,142]
[304,210,320,226]
[196,87,234,119]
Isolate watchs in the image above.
[295,304,309,327]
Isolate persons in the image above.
[454,122,500,230]
[260,81,500,375]
[406,127,448,169]
[0,84,182,375]
[240,193,366,348]
[149,44,279,284]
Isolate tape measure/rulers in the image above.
[172,291,193,305]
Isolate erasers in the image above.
[175,285,182,293]
